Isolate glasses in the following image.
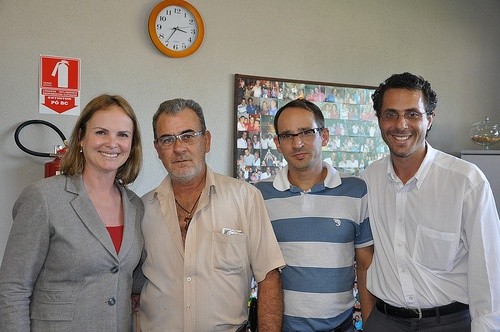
[277,128,319,144]
[378,110,429,121]
[157,132,202,148]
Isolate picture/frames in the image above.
[232,73,390,185]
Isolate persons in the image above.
[250,97,374,332]
[237,79,389,331]
[360,73,500,332]
[139,98,286,332]
[0,93,147,332]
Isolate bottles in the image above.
[470,114,500,150]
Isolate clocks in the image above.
[147,0,205,58]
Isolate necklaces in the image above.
[175,195,201,231]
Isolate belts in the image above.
[375,298,465,318]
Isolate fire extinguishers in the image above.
[43,146,67,178]
